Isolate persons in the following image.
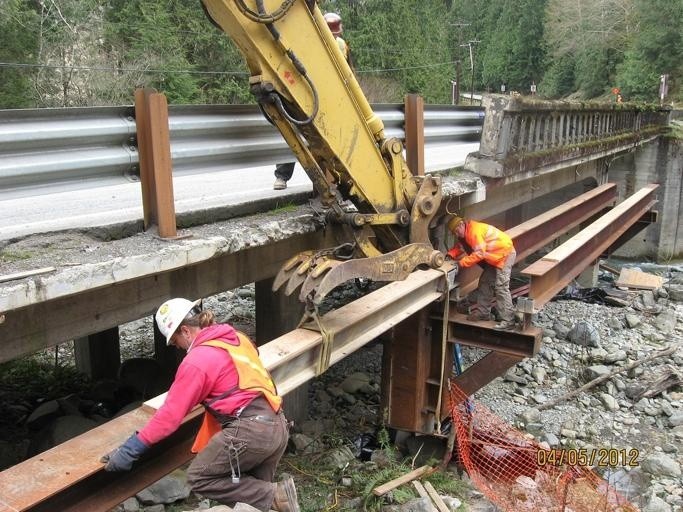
[617,95,624,103]
[97,297,301,511]
[443,216,517,331]
[325,13,358,81]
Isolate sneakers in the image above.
[494,321,515,330]
[271,479,299,512]
[467,313,489,321]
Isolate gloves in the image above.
[99,431,151,477]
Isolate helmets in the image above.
[155,298,202,347]
[324,12,342,35]
[448,217,464,232]
